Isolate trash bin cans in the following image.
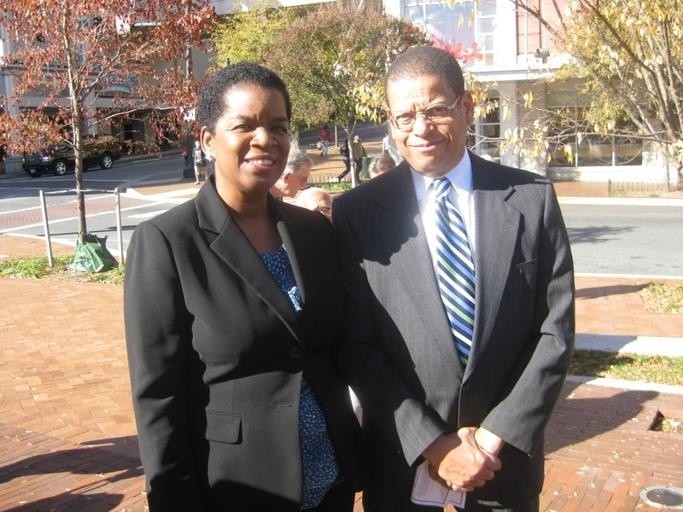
[361,157,372,176]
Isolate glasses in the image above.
[388,96,461,130]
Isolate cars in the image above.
[16,129,125,178]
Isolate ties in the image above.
[431,177,475,369]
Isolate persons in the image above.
[338,135,368,183]
[270,148,312,202]
[331,46,576,512]
[125,61,379,512]
[370,152,395,178]
[381,130,400,166]
[191,140,209,185]
[320,124,333,159]
[296,187,333,218]
